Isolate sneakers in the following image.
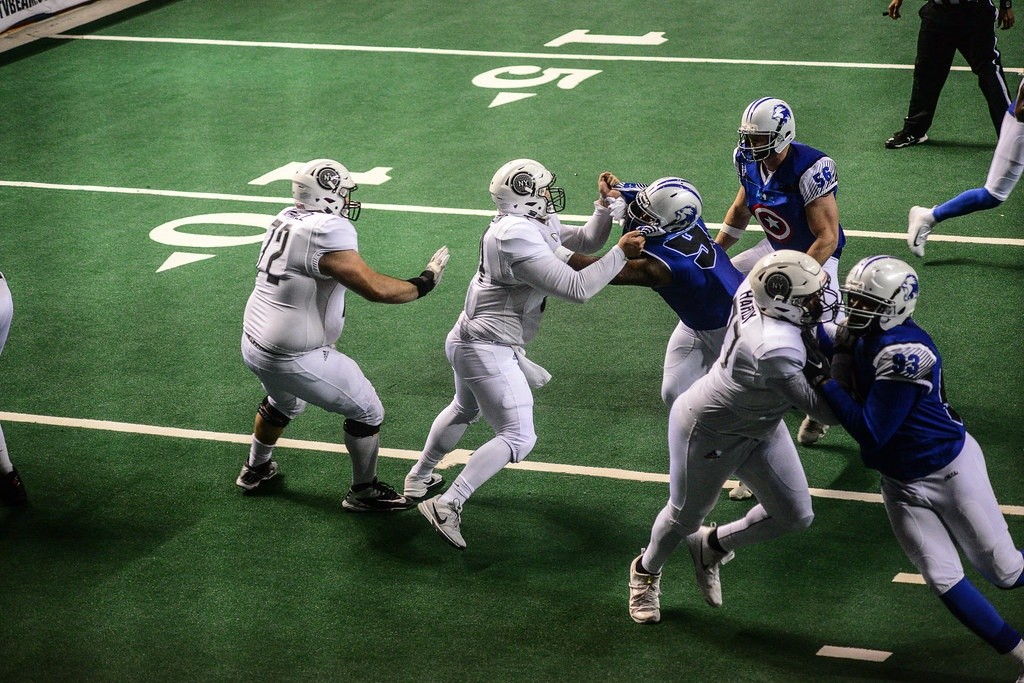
[342,476,414,512]
[729,483,754,500]
[418,494,466,550]
[236,454,278,491]
[628,547,662,623]
[687,522,729,607]
[885,131,928,149]
[403,472,443,499]
[798,415,829,445]
[0,466,27,505]
[907,206,934,257]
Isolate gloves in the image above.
[605,196,628,227]
[527,218,575,264]
[801,331,832,390]
[420,245,450,292]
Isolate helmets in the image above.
[737,97,796,162]
[489,159,567,219]
[748,250,838,327]
[833,255,918,330]
[626,177,703,236]
[293,159,361,222]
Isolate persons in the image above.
[885,0,1015,149]
[404,97,846,549]
[236,159,450,513]
[0,270,28,505]
[908,70,1024,257]
[629,248,1024,683]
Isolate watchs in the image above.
[999,1,1012,9]
